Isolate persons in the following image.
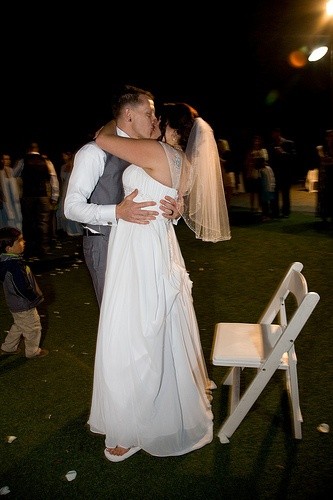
[63,85,185,311]
[1,225,50,359]
[0,140,84,251]
[216,125,333,225]
[87,102,232,463]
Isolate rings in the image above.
[169,210,174,216]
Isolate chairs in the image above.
[212,262,321,439]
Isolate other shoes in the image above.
[0,347,21,355]
[104,445,142,462]
[25,348,49,358]
